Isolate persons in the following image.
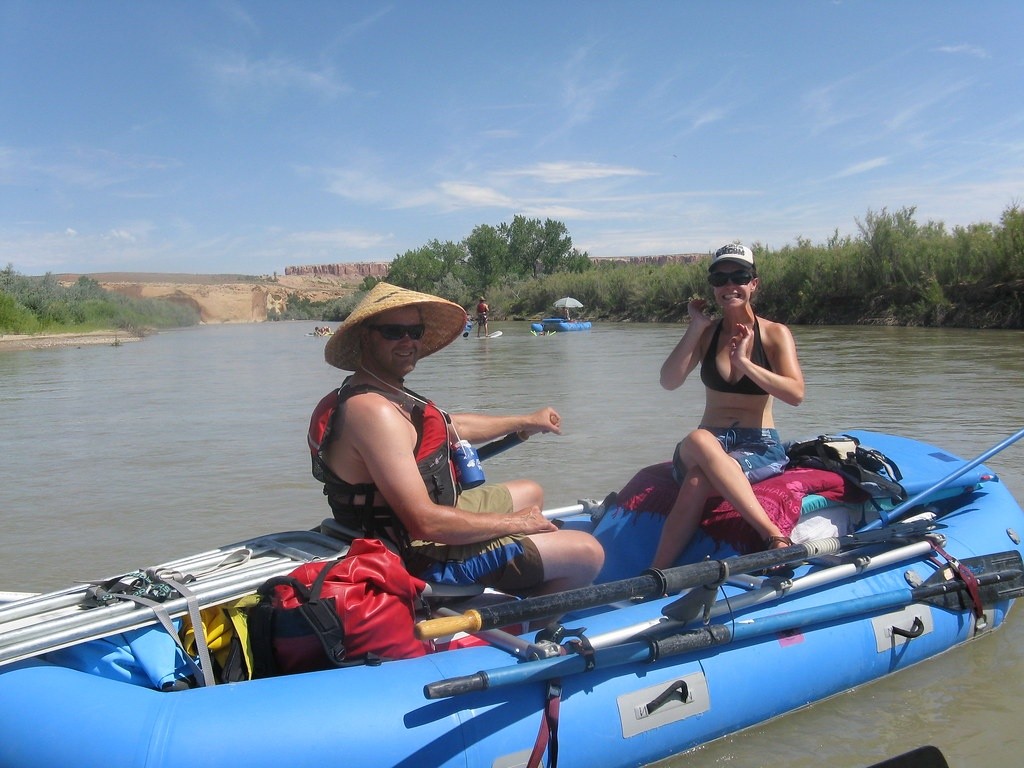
[308,282,605,638]
[629,244,804,603]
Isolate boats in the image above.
[531,318,592,334]
[0,429,1024,767]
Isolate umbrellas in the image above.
[553,297,584,307]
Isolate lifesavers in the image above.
[531,330,556,337]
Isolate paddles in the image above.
[414,519,948,641]
[303,414,558,533]
[463,320,478,337]
[424,551,1023,701]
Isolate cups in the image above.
[453,439,485,491]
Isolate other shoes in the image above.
[764,537,804,574]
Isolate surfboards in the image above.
[475,331,503,339]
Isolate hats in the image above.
[324,281,467,371]
[479,296,486,301]
[708,243,754,273]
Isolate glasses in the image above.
[708,269,756,288]
[367,323,426,341]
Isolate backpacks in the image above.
[780,433,909,507]
[220,540,435,684]
